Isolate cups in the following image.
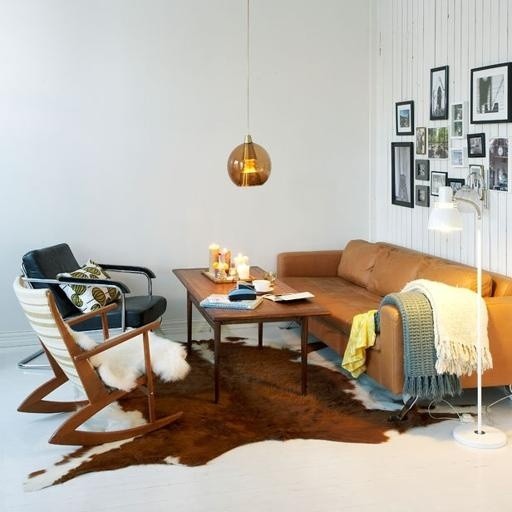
[207,243,249,280]
[253,280,270,291]
[263,271,279,288]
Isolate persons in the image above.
[479,76,493,114]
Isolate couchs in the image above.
[275,237,512,398]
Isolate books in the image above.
[263,292,315,302]
[200,293,264,310]
[229,289,256,301]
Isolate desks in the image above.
[171,265,334,405]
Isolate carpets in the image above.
[22,333,494,492]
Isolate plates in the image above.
[254,287,274,294]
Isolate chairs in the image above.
[12,273,185,446]
[16,241,168,372]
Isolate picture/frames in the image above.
[448,101,465,139]
[431,170,448,198]
[390,141,414,209]
[395,100,414,136]
[467,133,485,157]
[448,179,464,192]
[470,61,512,125]
[429,64,449,121]
[415,159,429,181]
[415,185,429,207]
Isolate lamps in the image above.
[227,0,273,189]
[426,184,507,450]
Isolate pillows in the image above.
[56,257,124,317]
